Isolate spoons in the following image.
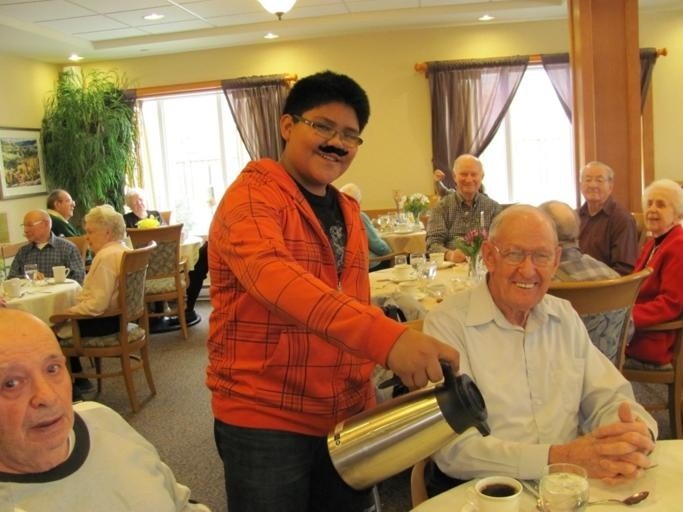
[585,489,650,508]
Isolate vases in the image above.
[411,210,425,228]
[463,255,486,284]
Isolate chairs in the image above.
[65,231,90,272]
[626,208,649,260]
[547,263,656,379]
[362,248,413,277]
[0,239,34,279]
[394,318,472,508]
[620,313,683,441]
[123,222,191,344]
[47,237,159,417]
[156,209,171,223]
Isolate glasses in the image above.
[290,114,362,149]
[488,240,553,268]
[19,220,43,228]
[58,199,75,207]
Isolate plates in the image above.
[411,292,424,300]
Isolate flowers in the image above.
[401,191,432,228]
[453,225,495,276]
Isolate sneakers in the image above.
[76,377,94,394]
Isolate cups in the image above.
[461,474,522,511]
[51,266,70,282]
[538,462,590,512]
[0,279,22,299]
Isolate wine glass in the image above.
[375,212,399,233]
[393,251,440,293]
[22,265,39,289]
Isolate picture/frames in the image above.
[0,125,49,201]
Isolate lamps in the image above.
[257,0,296,25]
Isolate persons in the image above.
[205,67,461,511]
[341,182,391,272]
[0,307,208,511]
[425,154,500,263]
[7,186,168,397]
[423,204,657,486]
[542,161,682,365]
